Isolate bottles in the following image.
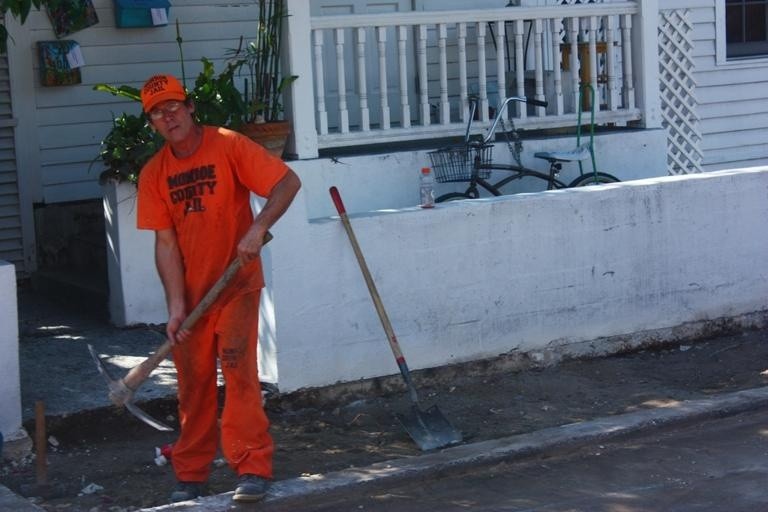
[417,166,435,208]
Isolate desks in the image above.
[557,39,620,131]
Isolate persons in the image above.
[138,75,304,502]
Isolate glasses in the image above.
[148,100,180,120]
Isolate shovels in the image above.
[330,187,461,450]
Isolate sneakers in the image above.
[171,480,207,502]
[232,473,268,501]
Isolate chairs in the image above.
[487,17,561,116]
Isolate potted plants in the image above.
[222,0,299,162]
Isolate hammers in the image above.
[21,400,60,500]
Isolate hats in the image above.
[141,74,187,113]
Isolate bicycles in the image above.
[425,91,621,204]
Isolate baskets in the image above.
[426,143,494,183]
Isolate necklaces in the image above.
[175,130,199,156]
